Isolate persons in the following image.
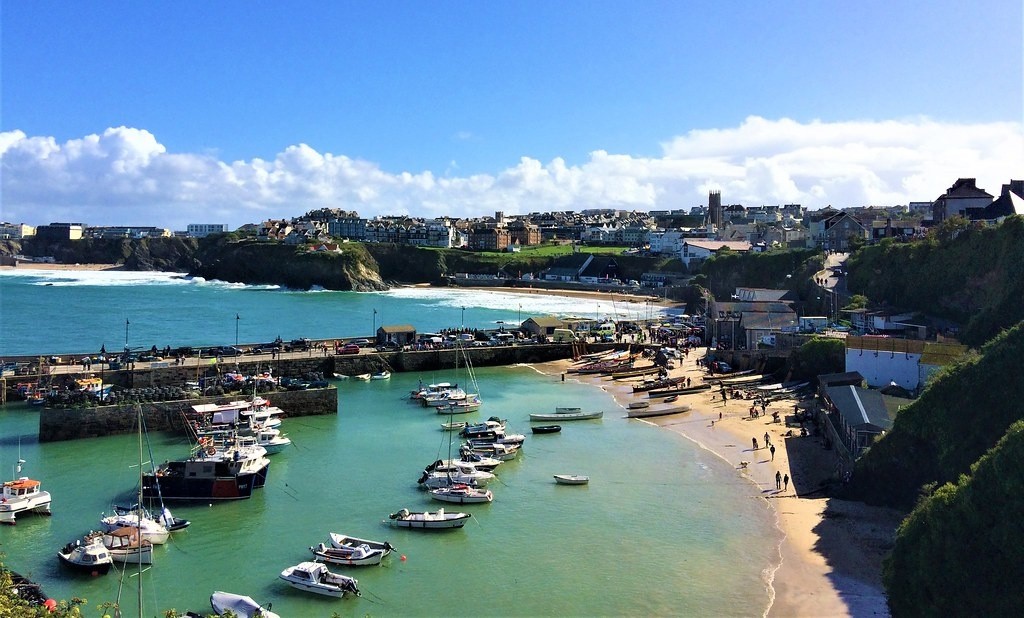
[776,471,789,490]
[752,432,775,460]
[588,318,702,348]
[410,328,563,351]
[313,343,327,352]
[772,405,820,437]
[209,343,310,362]
[69,345,186,370]
[712,380,772,425]
[642,348,720,389]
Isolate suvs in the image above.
[344,338,370,348]
[285,338,311,353]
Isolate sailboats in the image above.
[416,405,495,489]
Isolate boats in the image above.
[553,474,590,485]
[530,410,604,421]
[410,332,483,415]
[566,348,664,379]
[55,379,291,577]
[309,542,385,567]
[627,404,693,418]
[279,560,362,599]
[329,532,397,557]
[332,372,350,381]
[530,425,561,435]
[719,372,778,387]
[382,508,471,529]
[648,383,712,399]
[440,422,466,431]
[371,370,391,380]
[702,368,756,383]
[429,484,493,503]
[6,382,51,408]
[209,590,281,618]
[629,401,649,409]
[632,375,686,394]
[1,433,53,526]
[354,372,372,381]
[664,395,679,403]
[425,415,527,474]
[756,379,811,395]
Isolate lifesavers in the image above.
[208,447,217,455]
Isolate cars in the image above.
[376,341,400,352]
[604,333,615,343]
[649,315,707,348]
[338,344,361,355]
[253,343,280,355]
[511,330,525,340]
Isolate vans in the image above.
[553,328,580,343]
[489,333,515,346]
[596,323,617,337]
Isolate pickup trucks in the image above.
[208,345,241,357]
